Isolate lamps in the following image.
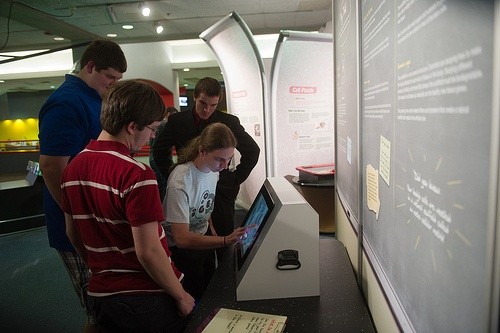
[151,20,164,34]
[137,2,151,17]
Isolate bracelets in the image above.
[223,235,227,247]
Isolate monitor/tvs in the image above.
[235,177,320,301]
[179,95,189,108]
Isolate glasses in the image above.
[145,125,159,135]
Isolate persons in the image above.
[64,80,195,333]
[149,77,261,299]
[38,39,127,333]
